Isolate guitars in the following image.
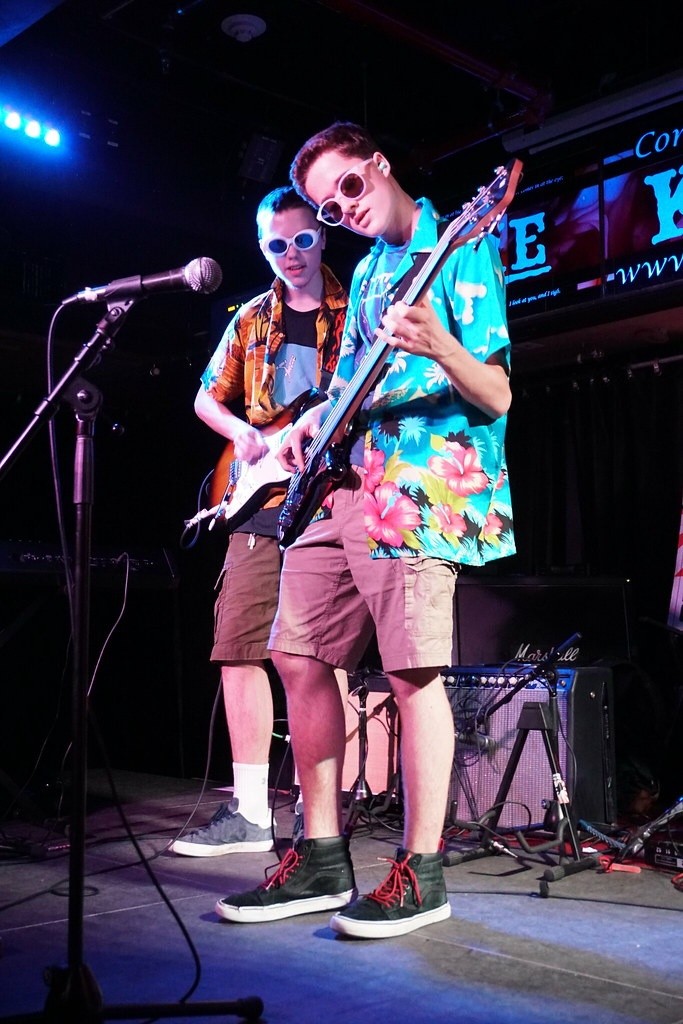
[204,386,330,539]
[275,156,524,555]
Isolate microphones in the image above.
[455,731,495,753]
[60,257,222,308]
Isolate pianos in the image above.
[0,536,182,591]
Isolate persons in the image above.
[214,123,518,940]
[167,184,348,859]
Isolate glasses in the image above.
[316,156,373,226]
[260,224,323,257]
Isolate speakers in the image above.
[339,662,613,837]
[450,569,635,669]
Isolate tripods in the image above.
[475,633,636,859]
[0,299,263,1024]
[342,665,398,838]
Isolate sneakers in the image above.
[167,804,275,857]
[291,813,305,842]
[330,838,451,938]
[214,834,358,922]
